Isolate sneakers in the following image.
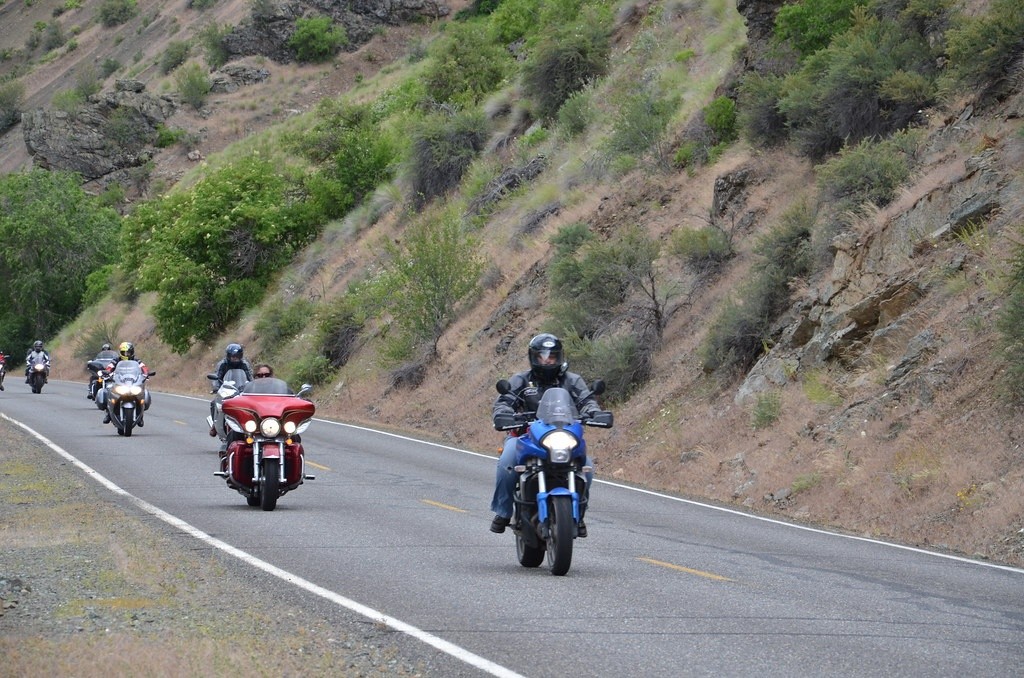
[490,515,509,533]
[578,521,587,537]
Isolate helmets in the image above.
[528,333,563,379]
[102,344,111,351]
[226,344,243,367]
[34,341,43,351]
[119,342,134,360]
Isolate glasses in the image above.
[256,373,271,378]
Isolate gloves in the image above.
[592,410,613,428]
[494,413,515,431]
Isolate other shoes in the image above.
[45,381,48,383]
[0,386,4,391]
[25,380,29,383]
[102,415,111,424]
[210,425,217,437]
[138,419,144,427]
[87,394,92,399]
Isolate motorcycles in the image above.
[87,350,156,436]
[496,378,613,575]
[206,369,249,439]
[0,355,9,389]
[26,356,51,394]
[212,380,316,511]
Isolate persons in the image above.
[0,351,4,363]
[87,344,111,399]
[209,343,254,437]
[25,340,50,383]
[490,333,603,538]
[97,342,148,427]
[223,363,293,433]
